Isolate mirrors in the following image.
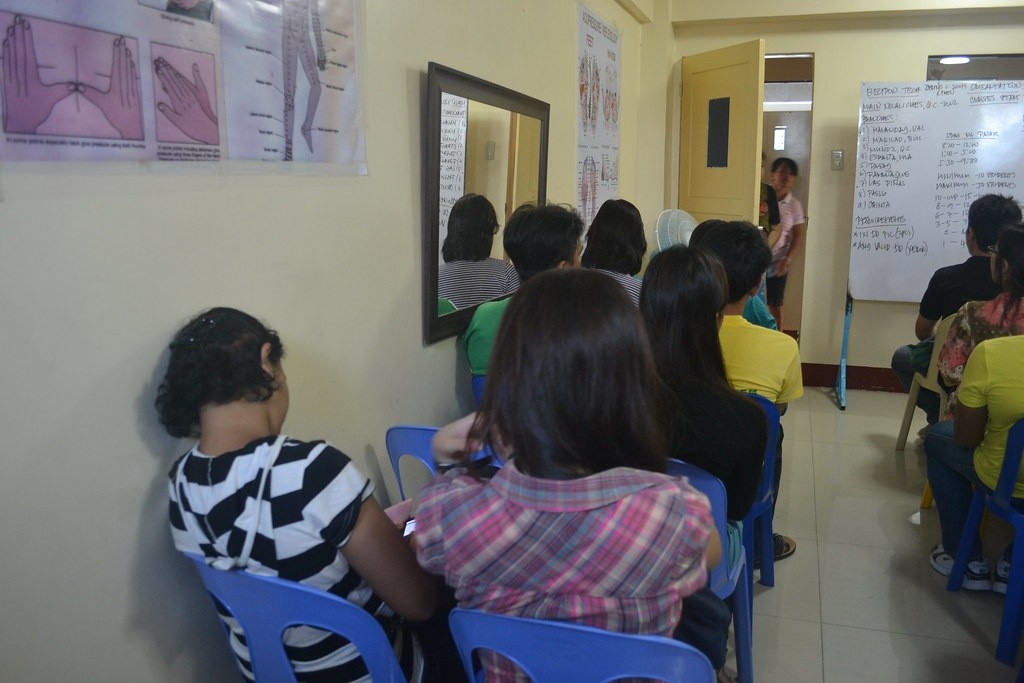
[415,57,553,351]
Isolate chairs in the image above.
[894,309,957,453]
[945,414,1024,668]
[383,423,502,500]
[446,602,720,683]
[741,388,781,592]
[664,457,757,683]
[174,548,406,683]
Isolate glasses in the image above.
[986,246,1000,256]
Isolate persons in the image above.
[891,193,1024,595]
[154,307,483,683]
[759,151,806,332]
[437,194,521,317]
[465,198,804,610]
[415,269,720,683]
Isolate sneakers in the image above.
[928,543,1010,595]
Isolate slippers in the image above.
[772,532,797,559]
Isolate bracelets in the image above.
[437,455,472,476]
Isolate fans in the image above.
[653,206,700,251]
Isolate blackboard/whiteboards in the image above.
[850,80,1024,302]
[440,93,468,285]
